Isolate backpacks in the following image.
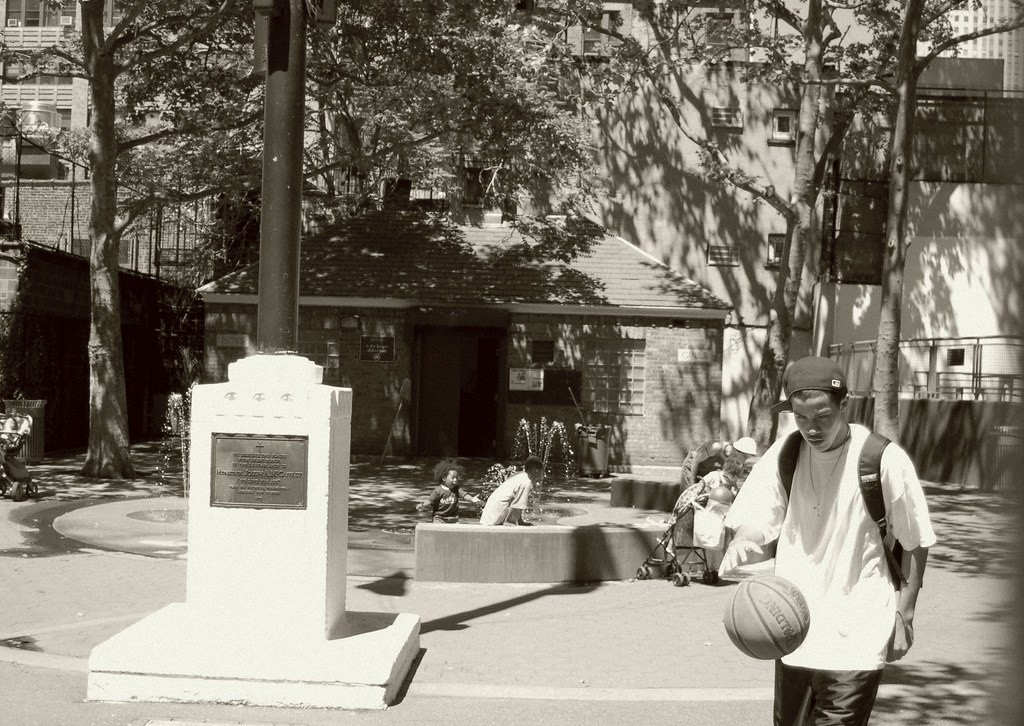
[778,429,905,591]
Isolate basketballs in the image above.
[722,573,809,660]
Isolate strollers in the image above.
[637,476,720,588]
[0,411,38,502]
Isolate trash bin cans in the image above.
[3,398,47,465]
[578,424,612,478]
[991,424,1023,492]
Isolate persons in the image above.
[416,462,481,524]
[678,437,758,490]
[479,455,544,525]
[717,357,938,726]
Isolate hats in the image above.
[733,436,756,456]
[770,356,848,412]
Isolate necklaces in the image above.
[809,428,850,518]
